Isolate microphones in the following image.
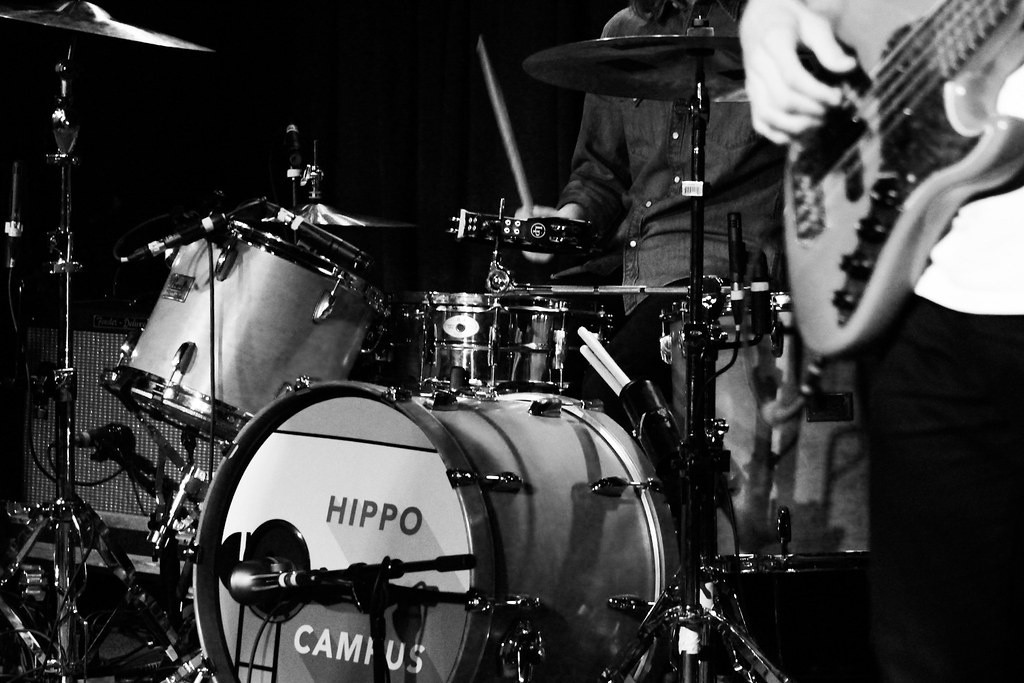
[8,159,21,268]
[265,202,376,274]
[286,124,302,178]
[231,563,308,601]
[726,211,745,331]
[54,425,122,448]
[748,249,777,403]
[122,212,228,263]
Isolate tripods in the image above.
[0,35,209,683]
[602,49,792,683]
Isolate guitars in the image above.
[778,1,1024,354]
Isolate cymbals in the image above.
[522,34,859,103]
[257,203,415,231]
[0,0,216,53]
[640,136,789,223]
[446,208,604,254]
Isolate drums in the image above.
[124,211,389,442]
[192,379,682,683]
[660,291,870,573]
[400,288,619,393]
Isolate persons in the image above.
[513,0,873,559]
[736,0,1024,683]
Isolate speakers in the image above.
[22,315,232,534]
